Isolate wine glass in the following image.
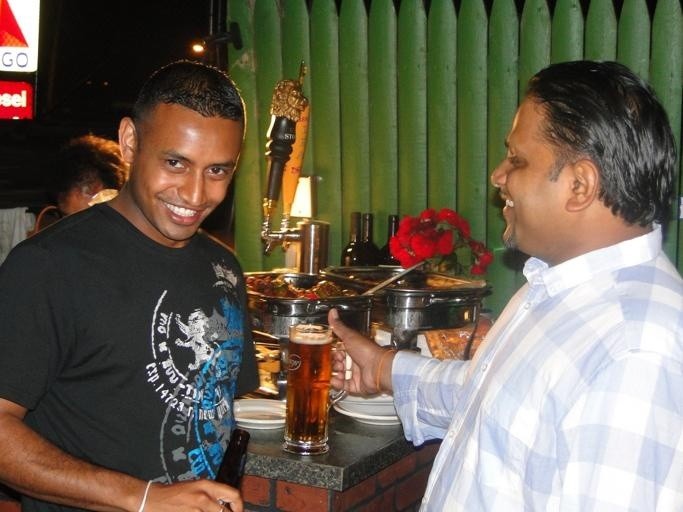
[216,497,224,512]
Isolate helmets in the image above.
[227,398,293,430]
[333,387,417,427]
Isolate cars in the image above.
[282,321,352,456]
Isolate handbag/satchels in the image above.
[138,479,151,512]
[374,346,395,395]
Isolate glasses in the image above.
[192,31,233,53]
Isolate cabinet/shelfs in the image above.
[343,211,361,267]
[361,213,380,266]
[379,214,404,267]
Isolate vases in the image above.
[388,207,493,276]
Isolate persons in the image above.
[53,135,129,216]
[0,61,248,512]
[327,61,682,511]
